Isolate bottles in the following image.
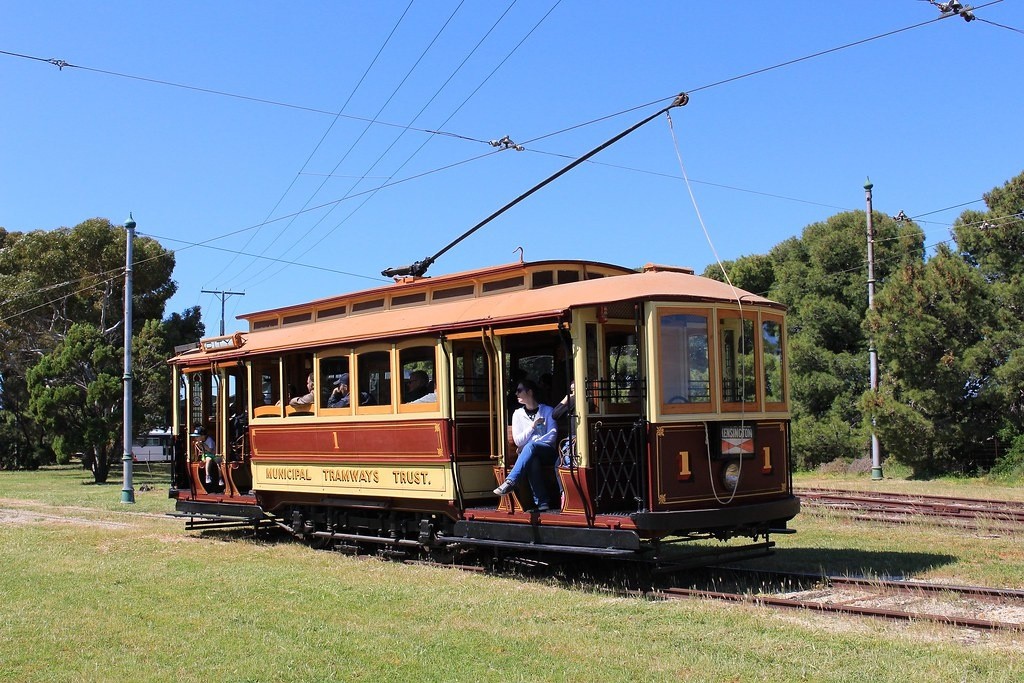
[536,424,545,437]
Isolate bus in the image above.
[168,91,803,574]
[132,426,176,463]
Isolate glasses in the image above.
[517,388,530,393]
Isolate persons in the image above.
[275,373,313,405]
[404,370,434,405]
[190,427,224,486]
[327,373,371,410]
[492,381,595,512]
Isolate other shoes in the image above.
[527,502,549,513]
[205,475,212,483]
[218,479,224,486]
[560,492,565,511]
[494,481,515,495]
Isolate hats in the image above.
[333,373,349,385]
[189,426,207,436]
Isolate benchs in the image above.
[507,425,557,513]
